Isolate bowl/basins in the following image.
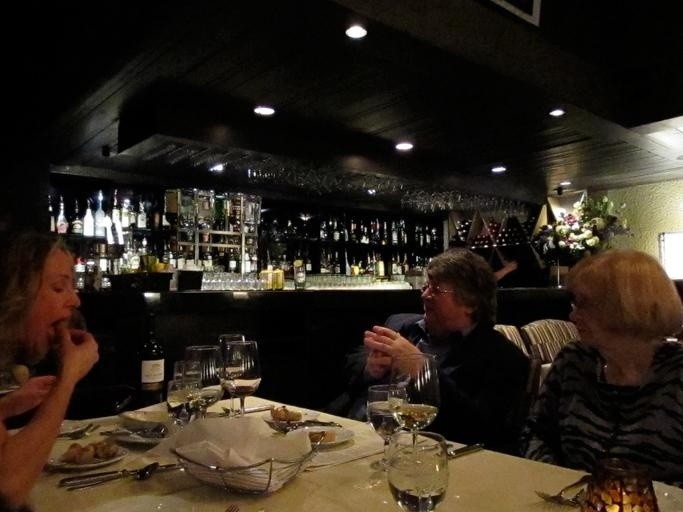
[121,411,169,429]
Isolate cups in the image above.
[261,270,273,291]
[293,262,306,290]
[306,275,375,288]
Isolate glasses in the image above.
[419,283,465,297]
[572,302,604,312]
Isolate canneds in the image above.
[258,271,273,290]
[350,265,360,276]
[375,260,385,277]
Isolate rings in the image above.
[392,331,402,341]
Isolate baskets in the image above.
[170,430,326,497]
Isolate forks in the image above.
[227,506,240,512]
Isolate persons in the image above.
[348,246,531,461]
[1,227,101,511]
[492,253,519,284]
[516,247,683,490]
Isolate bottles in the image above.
[365,254,373,275]
[319,218,440,245]
[127,211,136,224]
[492,227,525,247]
[335,252,341,273]
[142,313,165,406]
[112,190,120,224]
[137,200,147,229]
[351,256,359,276]
[84,201,93,237]
[488,222,500,239]
[178,245,185,267]
[228,250,237,272]
[390,255,431,275]
[185,247,195,269]
[520,216,537,235]
[370,253,377,275]
[342,251,351,275]
[95,190,104,236]
[47,195,55,232]
[202,247,212,269]
[73,237,178,274]
[57,196,70,233]
[455,219,472,241]
[468,232,493,250]
[71,200,83,237]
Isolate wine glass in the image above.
[367,385,402,471]
[223,341,262,416]
[219,333,244,414]
[391,431,447,512]
[182,346,222,418]
[201,271,260,291]
[400,189,525,217]
[388,357,440,447]
[174,359,203,394]
[169,381,200,433]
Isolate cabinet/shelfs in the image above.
[40,162,153,276]
[163,186,262,276]
[445,207,546,268]
[258,196,444,274]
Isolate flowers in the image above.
[537,195,634,257]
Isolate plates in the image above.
[113,429,172,446]
[295,426,357,448]
[45,445,128,472]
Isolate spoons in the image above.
[69,464,160,489]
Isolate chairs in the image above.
[493,323,543,401]
[521,317,582,364]
